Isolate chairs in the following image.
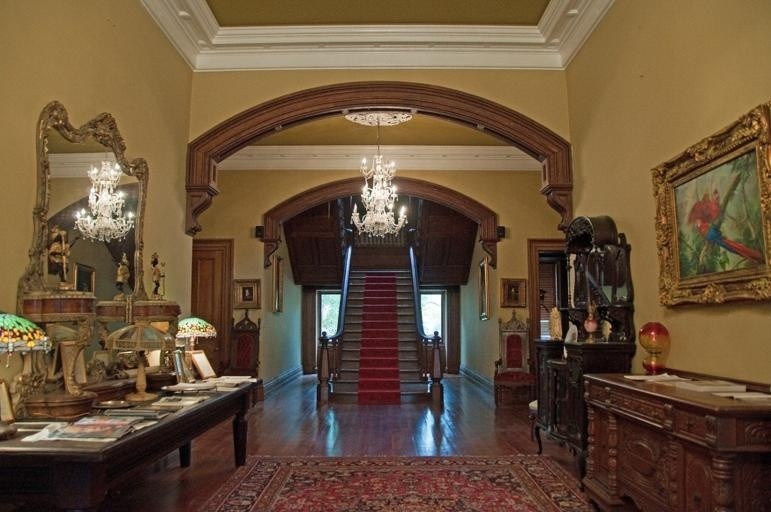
[528,400,539,440]
[217,309,260,408]
[495,311,539,408]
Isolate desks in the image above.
[0,376,265,512]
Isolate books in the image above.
[21,396,207,445]
[622,373,771,402]
[173,349,217,384]
[161,374,257,395]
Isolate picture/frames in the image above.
[272,254,284,313]
[477,256,490,321]
[651,100,771,311]
[72,262,96,293]
[190,350,217,380]
[500,277,527,307]
[172,349,187,384]
[59,340,87,392]
[232,278,262,309]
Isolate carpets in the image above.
[197,455,600,512]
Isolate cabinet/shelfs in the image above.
[582,366,771,512]
[532,340,637,491]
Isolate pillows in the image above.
[343,108,413,239]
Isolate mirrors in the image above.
[16,99,150,301]
[566,210,634,309]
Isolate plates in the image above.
[90,400,136,409]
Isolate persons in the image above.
[150,252,164,296]
[115,253,130,294]
[243,288,252,300]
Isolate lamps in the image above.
[638,322,671,375]
[176,316,217,378]
[105,320,175,402]
[0,310,53,439]
